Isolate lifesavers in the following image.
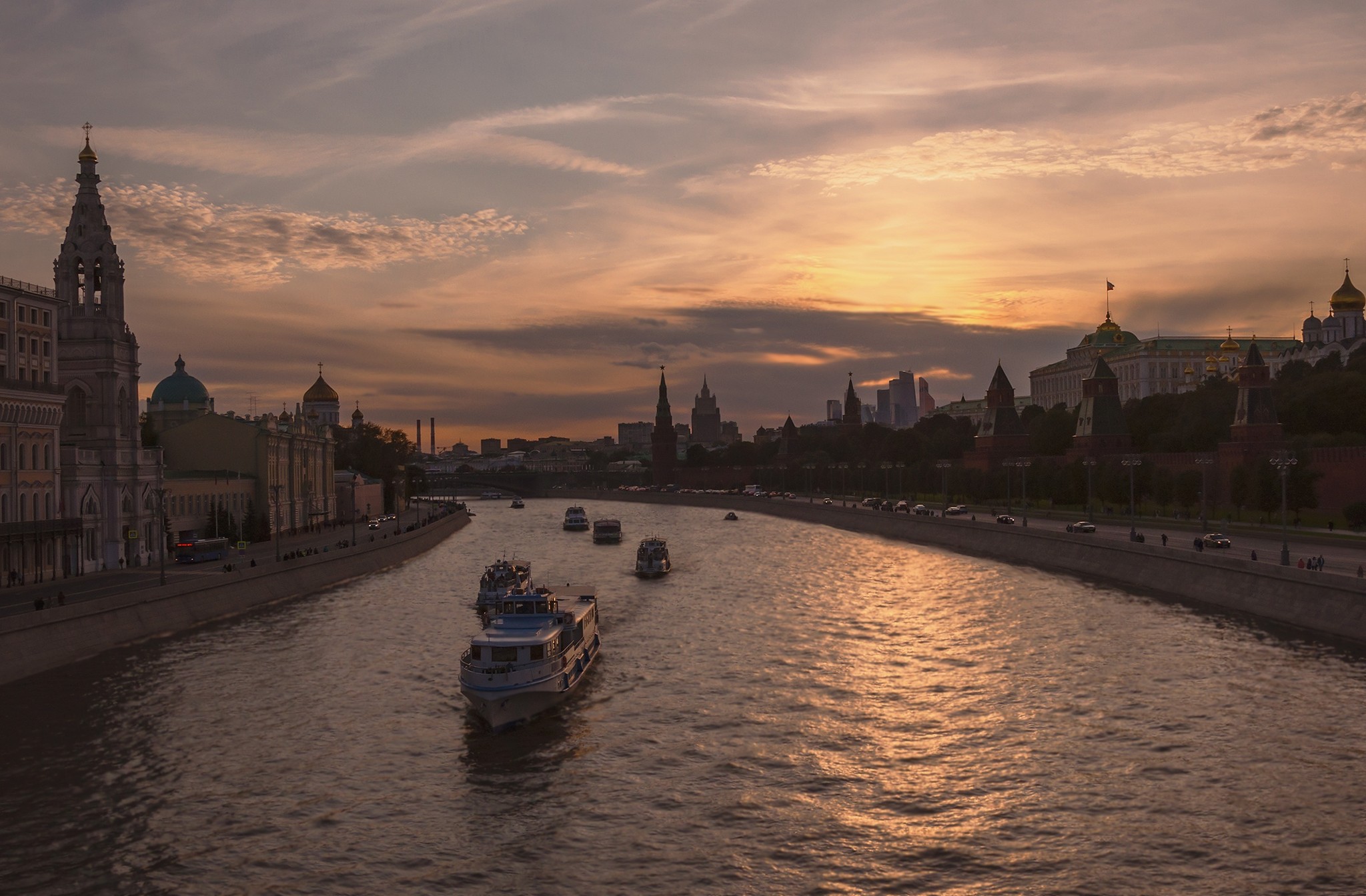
[565,613,573,625]
[482,611,490,625]
[639,548,645,555]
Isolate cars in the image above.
[369,514,396,530]
[619,486,658,492]
[1074,522,1096,533]
[862,498,925,511]
[660,488,796,499]
[946,505,968,515]
[997,515,1014,524]
[822,497,832,505]
[1204,532,1231,547]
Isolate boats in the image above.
[458,585,604,734]
[511,497,525,508]
[480,492,502,500]
[593,518,623,545]
[725,511,737,521]
[635,537,672,578]
[474,559,533,613]
[563,506,589,530]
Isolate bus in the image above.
[174,537,230,565]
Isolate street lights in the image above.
[1121,455,1141,542]
[896,461,905,499]
[1196,459,1214,532]
[936,460,952,510]
[1001,457,1032,528]
[413,477,423,528]
[732,462,867,507]
[391,479,404,535]
[149,488,173,586]
[428,476,458,517]
[269,484,284,562]
[1083,456,1097,523]
[1270,449,1299,565]
[880,461,893,500]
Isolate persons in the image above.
[1329,519,1334,533]
[223,564,235,573]
[1228,511,1232,524]
[1259,517,1265,531]
[468,508,470,512]
[34,592,65,610]
[1251,550,1257,561]
[1297,555,1325,571]
[135,554,140,566]
[11,568,18,586]
[283,499,464,561]
[18,570,21,586]
[251,558,256,567]
[148,553,152,566]
[119,557,124,570]
[500,573,505,587]
[872,504,1204,553]
[1294,519,1297,530]
[1358,565,1363,578]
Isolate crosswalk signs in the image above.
[237,541,246,549]
[128,531,137,539]
